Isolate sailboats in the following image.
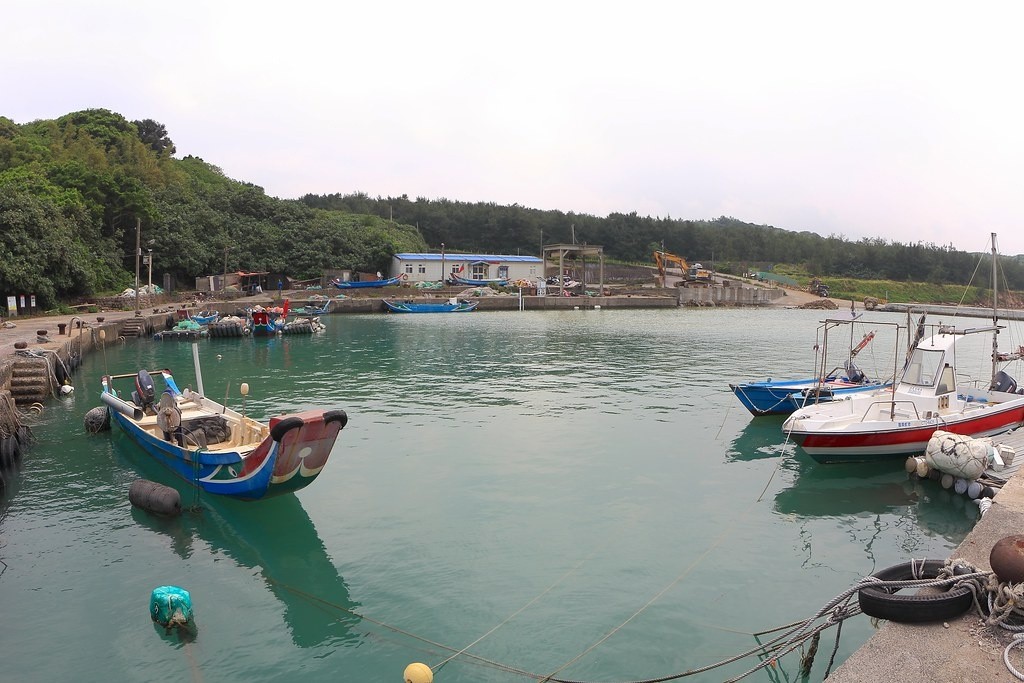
[779,233,1024,463]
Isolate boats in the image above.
[728,300,900,417]
[450,273,510,286]
[99,367,349,501]
[331,272,404,288]
[285,276,324,286]
[154,305,328,340]
[382,298,480,313]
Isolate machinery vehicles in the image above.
[653,250,717,285]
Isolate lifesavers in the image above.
[0,435,22,469]
[286,323,313,333]
[859,559,981,622]
[210,322,243,336]
[403,274,408,280]
